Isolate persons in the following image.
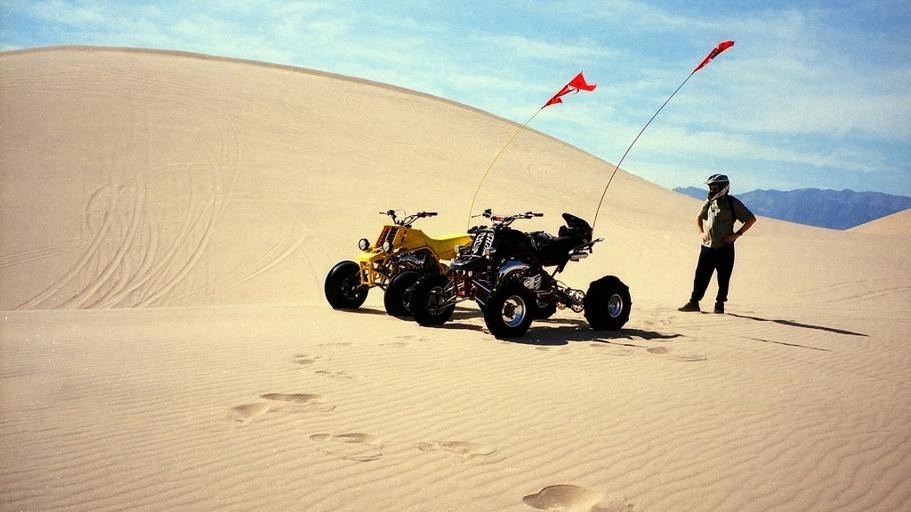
[677,175,756,315]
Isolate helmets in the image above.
[705,173,731,201]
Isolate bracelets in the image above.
[736,231,743,235]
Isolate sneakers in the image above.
[714,302,725,314]
[678,299,701,312]
